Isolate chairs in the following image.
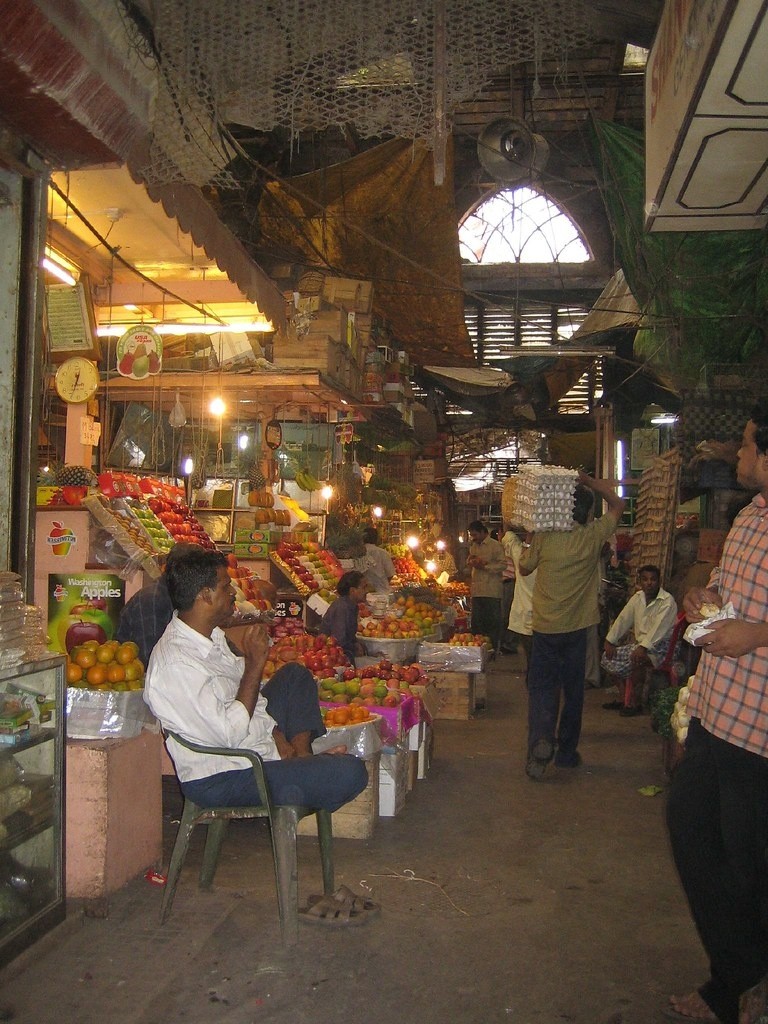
[628,611,687,709]
[159,726,332,952]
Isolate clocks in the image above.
[54,357,99,403]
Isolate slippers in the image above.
[298,898,368,927]
[307,884,382,916]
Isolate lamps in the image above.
[96,318,273,335]
[614,439,625,498]
[237,426,250,450]
[651,413,678,424]
[210,336,226,414]
[322,401,333,501]
[182,449,194,476]
[42,239,83,286]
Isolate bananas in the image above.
[296,467,323,492]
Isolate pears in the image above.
[119,341,161,378]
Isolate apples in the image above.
[57,594,113,654]
[131,495,272,616]
[255,633,428,706]
[449,632,493,650]
[277,541,469,639]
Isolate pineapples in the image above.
[241,447,267,489]
[190,445,209,489]
[37,465,99,487]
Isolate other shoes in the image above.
[603,699,623,710]
[619,705,643,717]
[555,752,581,768]
[500,644,518,654]
[525,738,555,779]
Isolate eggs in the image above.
[513,480,574,528]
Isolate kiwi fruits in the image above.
[105,508,154,557]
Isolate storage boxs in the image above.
[663,734,686,777]
[271,261,444,438]
[34,442,489,839]
[682,363,768,591]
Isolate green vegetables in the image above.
[649,683,684,728]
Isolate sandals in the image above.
[661,992,721,1024]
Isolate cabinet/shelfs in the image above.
[0,652,69,965]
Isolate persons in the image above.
[466,521,522,654]
[502,476,626,779]
[664,416,768,1024]
[601,565,681,718]
[115,543,244,673]
[142,552,369,811]
[363,528,396,602]
[318,572,380,666]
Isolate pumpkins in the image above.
[248,490,290,526]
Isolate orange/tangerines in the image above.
[320,704,374,727]
[64,639,146,692]
